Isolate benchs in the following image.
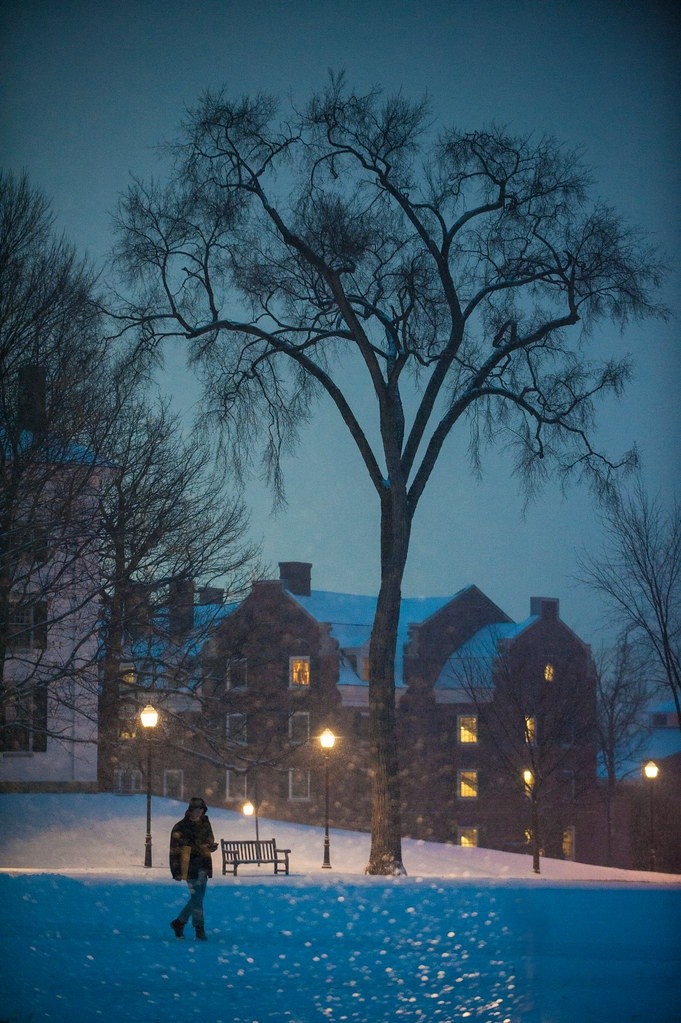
[220,838,290,877]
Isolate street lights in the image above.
[643,760,659,872]
[140,703,159,868]
[319,727,335,869]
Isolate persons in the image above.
[170,797,218,940]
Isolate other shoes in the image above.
[171,918,185,940]
[196,927,207,940]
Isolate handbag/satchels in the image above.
[179,844,191,881]
